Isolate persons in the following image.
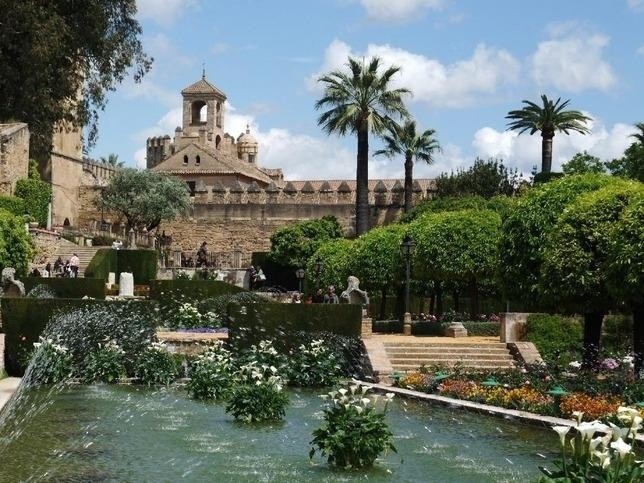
[253,263,267,289]
[196,240,210,267]
[70,251,80,277]
[54,256,64,271]
[324,284,339,303]
[312,287,324,302]
[249,264,257,291]
[303,292,313,304]
[62,259,70,275]
[46,262,51,277]
[111,237,123,250]
[291,292,301,303]
